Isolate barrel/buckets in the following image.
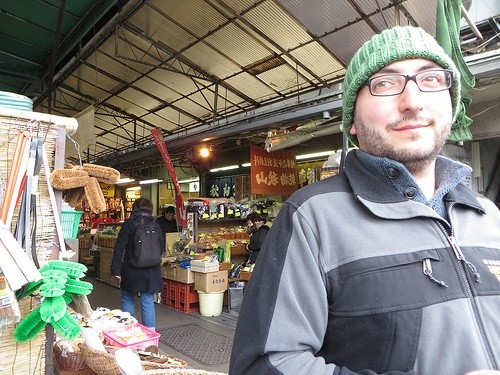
[197,289,225,317]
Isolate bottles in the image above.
[197,225,247,263]
[174,238,184,252]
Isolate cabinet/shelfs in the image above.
[82,198,134,227]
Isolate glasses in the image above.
[366,70,454,97]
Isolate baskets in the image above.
[77,342,188,375]
[60,211,84,240]
[51,342,89,371]
[143,370,228,375]
[103,322,161,350]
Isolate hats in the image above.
[342,26,462,147]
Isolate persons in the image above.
[241,212,272,267]
[229,25,500,375]
[155,206,178,252]
[111,197,163,331]
[90,210,120,230]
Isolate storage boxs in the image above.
[160,257,252,314]
[64,237,79,262]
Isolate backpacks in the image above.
[130,217,162,268]
